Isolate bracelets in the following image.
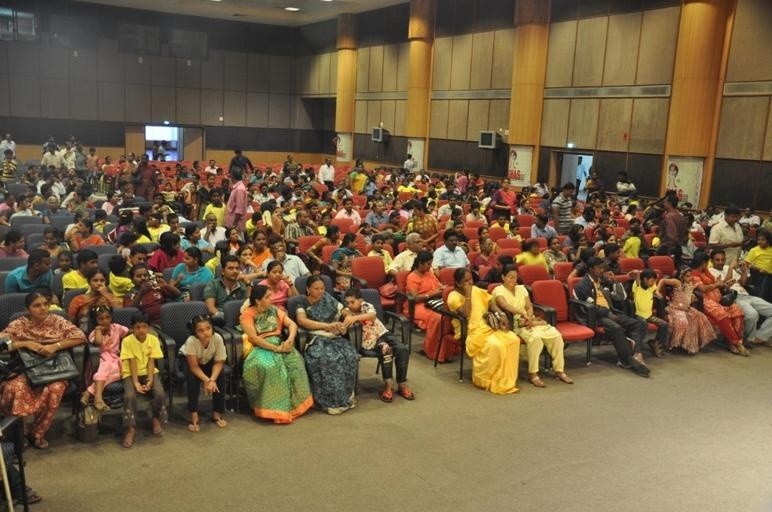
[96,328,102,330]
[424,293,429,300]
[465,295,472,300]
[709,283,713,290]
[56,342,62,350]
[273,345,278,353]
[499,312,506,316]
[286,340,293,346]
[358,314,360,321]
[208,379,216,382]
[742,269,747,273]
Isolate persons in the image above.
[107,254,136,300]
[330,231,365,295]
[708,203,752,275]
[123,263,182,325]
[79,304,160,414]
[250,231,272,266]
[391,232,424,278]
[6,248,53,294]
[61,135,126,258]
[1,290,88,449]
[491,265,573,387]
[126,141,165,246]
[759,207,772,236]
[256,260,301,313]
[202,255,249,325]
[654,265,717,355]
[261,237,313,286]
[627,268,668,359]
[226,163,248,245]
[313,157,361,235]
[541,236,567,278]
[61,249,99,292]
[508,150,519,171]
[1,465,41,505]
[571,255,651,378]
[293,275,359,415]
[739,222,757,251]
[485,254,523,285]
[176,314,229,432]
[707,249,772,349]
[52,250,74,276]
[367,234,393,275]
[119,311,165,448]
[683,199,714,250]
[147,232,187,274]
[570,156,662,254]
[513,241,549,275]
[224,227,247,257]
[465,263,480,281]
[412,202,439,253]
[431,228,471,278]
[568,255,629,262]
[67,268,123,324]
[739,205,761,229]
[657,193,689,279]
[126,245,147,266]
[403,250,456,363]
[237,244,268,279]
[165,140,179,232]
[414,168,555,209]
[34,285,64,311]
[248,168,272,239]
[342,287,416,401]
[170,246,214,302]
[305,224,342,272]
[568,248,596,280]
[272,154,313,244]
[665,163,679,192]
[688,251,750,356]
[431,210,553,241]
[601,243,630,276]
[447,267,521,393]
[361,155,414,239]
[229,149,255,175]
[469,236,500,274]
[1,130,62,257]
[551,182,576,235]
[741,228,771,304]
[179,144,230,251]
[239,285,315,424]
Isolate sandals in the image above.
[188,422,199,432]
[32,436,49,449]
[398,389,414,400]
[123,429,135,448]
[152,424,163,437]
[528,376,545,387]
[555,373,574,384]
[648,338,662,358]
[213,417,226,427]
[80,392,88,406]
[381,390,393,402]
[94,401,111,414]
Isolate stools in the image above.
[1,413,30,512]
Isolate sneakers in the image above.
[617,360,631,368]
[17,493,42,505]
[736,344,750,357]
[728,345,739,354]
[629,355,650,376]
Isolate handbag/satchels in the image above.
[425,298,443,312]
[719,290,737,306]
[16,346,80,386]
[484,311,501,331]
